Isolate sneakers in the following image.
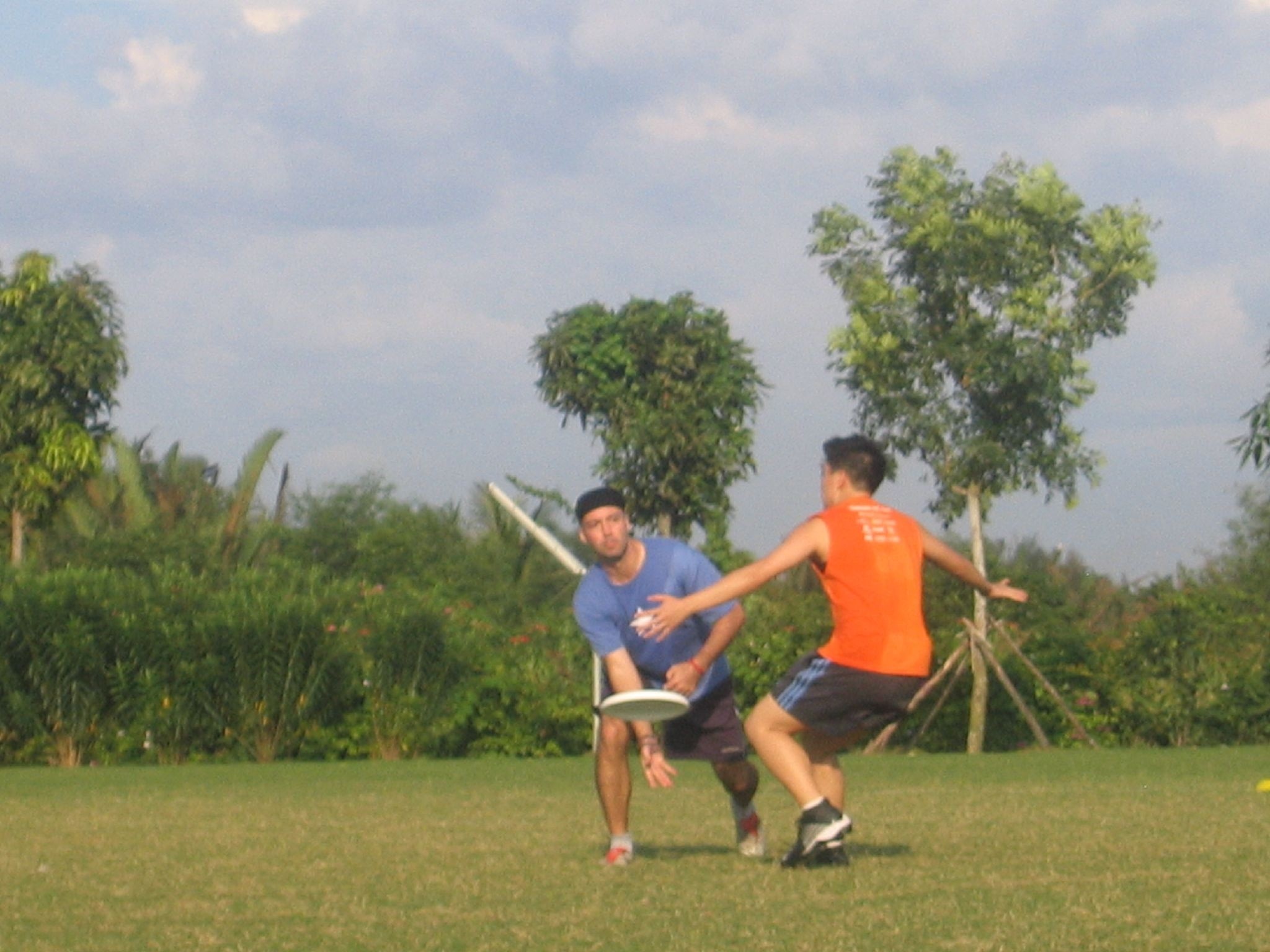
[736,811,765,857]
[780,798,853,867]
[606,847,632,867]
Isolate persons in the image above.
[631,433,1031,866]
[570,487,765,867]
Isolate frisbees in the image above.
[598,689,691,723]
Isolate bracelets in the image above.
[636,734,659,749]
[688,657,705,677]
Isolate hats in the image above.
[575,487,624,523]
